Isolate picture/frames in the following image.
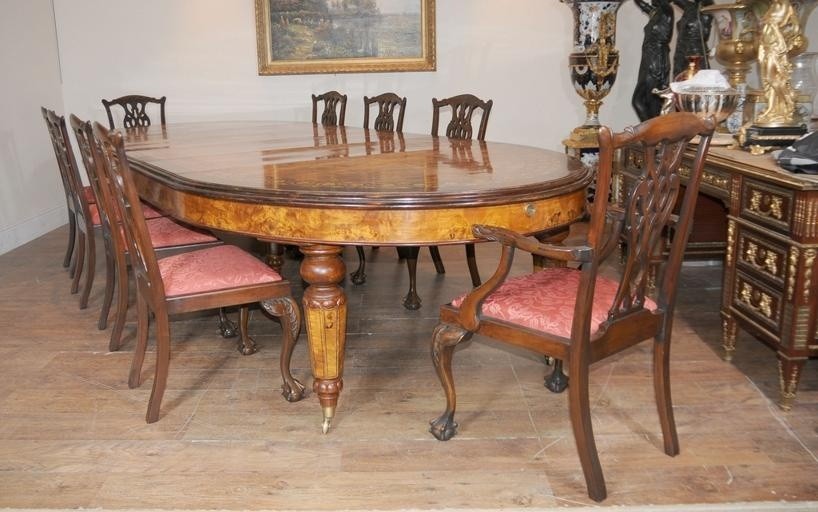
[255,0,436,75]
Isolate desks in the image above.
[620,128,818,413]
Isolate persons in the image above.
[630,0,675,124]
[671,0,715,82]
